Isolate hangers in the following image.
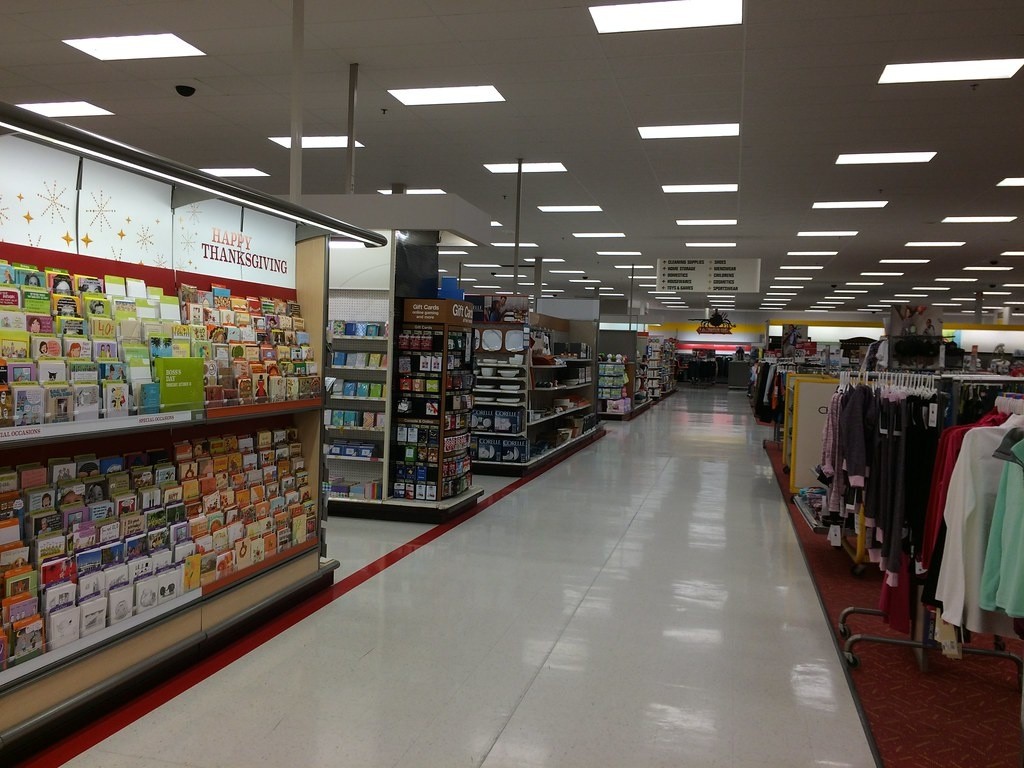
[762,355,1023,446]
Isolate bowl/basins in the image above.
[483,358,497,363]
[474,366,519,378]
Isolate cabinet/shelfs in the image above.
[384,323,485,525]
[523,358,607,477]
[632,330,678,415]
[0,98,388,757]
[324,334,390,521]
[469,320,528,477]
[597,361,635,421]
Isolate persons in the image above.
[748,357,757,398]
[788,325,801,346]
[500,296,507,304]
[923,319,934,335]
[737,347,744,359]
[489,299,507,322]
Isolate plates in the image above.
[474,397,495,401]
[475,329,525,352]
[498,360,508,364]
[496,398,520,403]
[500,385,520,390]
[475,385,495,389]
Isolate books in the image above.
[0,259,318,673]
